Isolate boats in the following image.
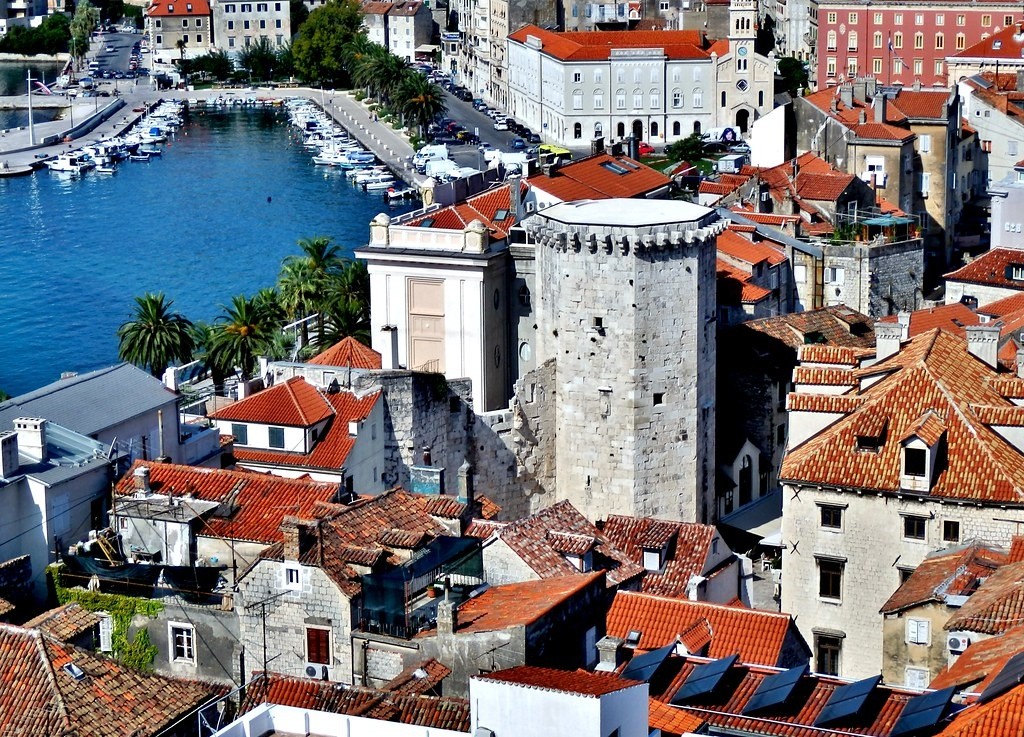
[382,186,416,200]
[43,95,398,189]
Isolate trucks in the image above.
[718,155,745,173]
[680,175,714,193]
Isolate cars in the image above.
[87,25,150,79]
[729,143,751,154]
[409,62,540,183]
[510,138,525,149]
[702,143,727,154]
[639,142,656,155]
[527,144,540,155]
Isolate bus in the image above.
[539,145,573,165]
